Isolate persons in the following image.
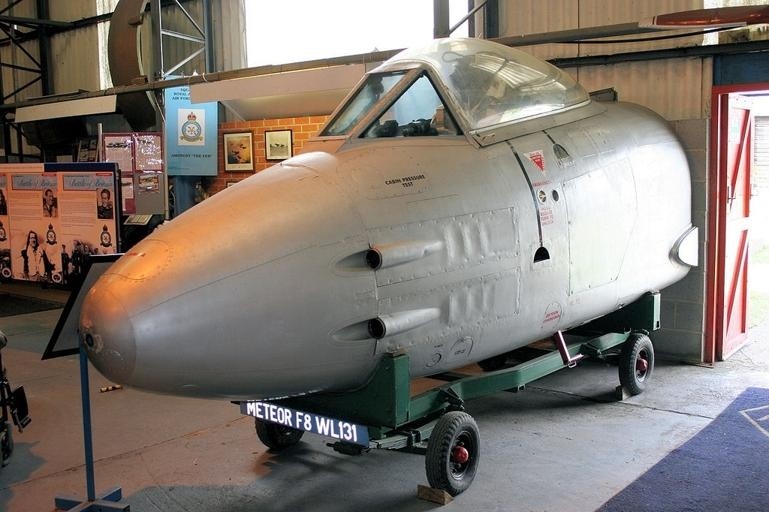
[97,189,114,220]
[43,189,57,216]
[19,230,99,285]
[0,189,7,215]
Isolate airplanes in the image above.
[82,31,700,401]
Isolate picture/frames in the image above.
[223,129,294,191]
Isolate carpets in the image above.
[597,386,769,511]
